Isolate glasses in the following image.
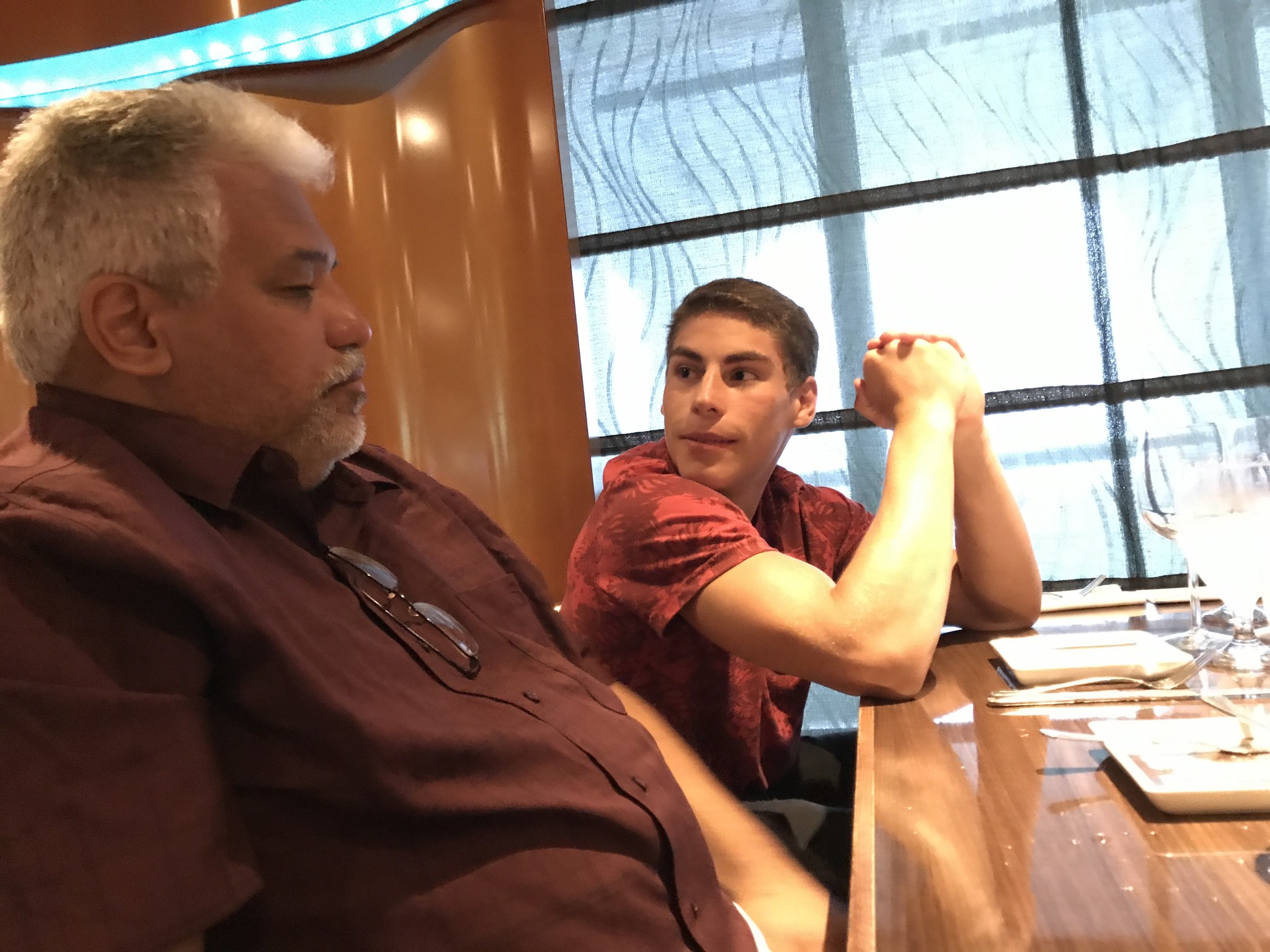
[325,548,482,681]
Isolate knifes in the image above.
[987,688,1270,707]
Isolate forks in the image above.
[1042,574,1107,598]
[990,649,1217,697]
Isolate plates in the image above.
[1088,716,1270,812]
[989,630,1194,688]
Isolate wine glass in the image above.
[1171,416,1270,674]
[1136,422,1233,650]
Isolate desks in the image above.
[842,595,1270,952]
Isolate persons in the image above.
[564,276,1042,907]
[1,82,850,952]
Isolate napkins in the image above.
[1039,585,1222,614]
